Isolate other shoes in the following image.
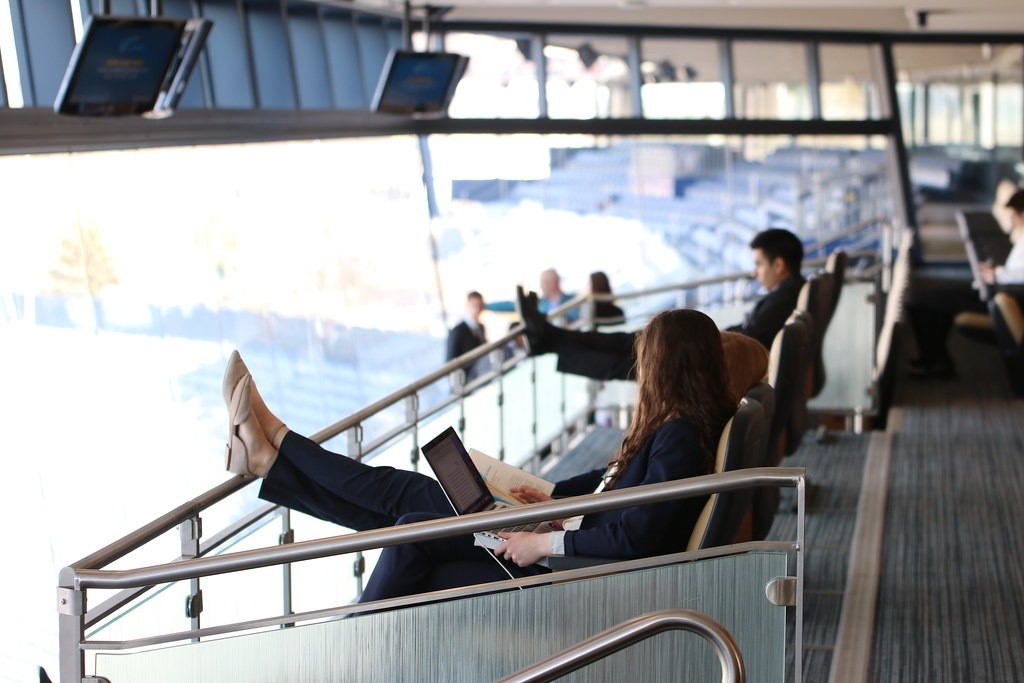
[516,286,548,356]
[226,374,278,479]
[528,291,548,321]
[909,354,922,366]
[907,367,957,380]
[223,350,286,446]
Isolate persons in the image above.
[221,229,805,613]
[977,145,1024,360]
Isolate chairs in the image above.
[685,247,847,547]
[949,207,1024,363]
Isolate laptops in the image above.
[421,425,559,543]
